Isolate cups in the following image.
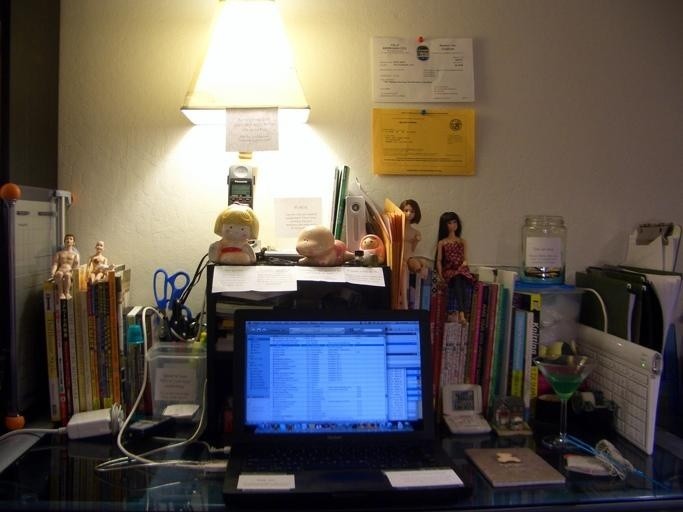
[520,214,569,285]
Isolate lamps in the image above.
[180,0,311,125]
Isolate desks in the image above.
[0,417,683,512]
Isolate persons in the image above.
[45,232,83,300]
[293,224,356,266]
[431,209,475,326]
[395,200,424,294]
[207,205,260,265]
[85,240,108,286]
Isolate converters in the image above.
[64,409,114,441]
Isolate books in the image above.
[415,261,541,442]
[44,262,153,428]
[361,195,414,310]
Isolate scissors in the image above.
[154,268,190,343]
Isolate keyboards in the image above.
[575,322,665,455]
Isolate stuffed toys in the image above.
[358,234,386,267]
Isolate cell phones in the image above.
[128,413,171,440]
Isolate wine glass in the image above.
[532,354,600,449]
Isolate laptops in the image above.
[221,307,472,503]
